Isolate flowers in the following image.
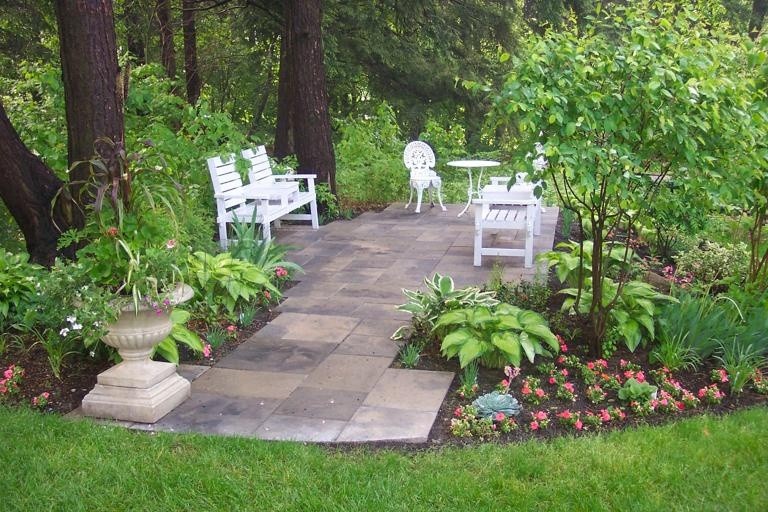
[19,130,196,362]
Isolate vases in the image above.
[70,283,195,425]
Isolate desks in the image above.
[446,160,500,218]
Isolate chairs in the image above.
[403,140,448,214]
[522,142,548,213]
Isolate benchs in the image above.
[471,170,542,268]
[205,144,320,252]
